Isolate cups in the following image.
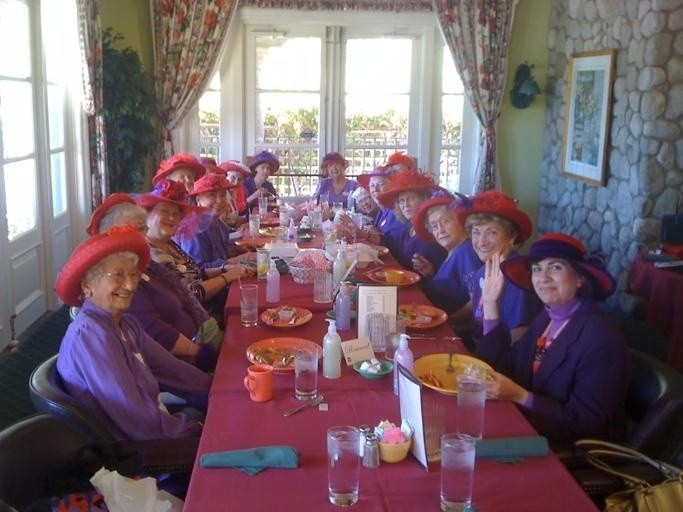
[326,426,361,506]
[458,382,486,441]
[239,194,420,327]
[383,316,406,363]
[295,345,319,399]
[440,432,477,511]
[245,363,273,403]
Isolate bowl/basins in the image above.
[353,359,393,380]
[380,439,412,463]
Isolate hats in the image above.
[135,181,212,219]
[56,228,150,309]
[87,192,137,235]
[321,152,346,166]
[455,190,532,242]
[500,233,619,300]
[357,150,449,209]
[413,191,454,245]
[152,150,252,196]
[251,152,280,173]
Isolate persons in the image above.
[153,151,281,270]
[480,233,631,476]
[55,225,214,476]
[351,152,483,348]
[135,178,259,328]
[448,190,538,369]
[312,152,358,210]
[85,191,224,374]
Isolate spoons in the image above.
[283,393,325,417]
[446,349,454,373]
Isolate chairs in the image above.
[27,354,202,473]
[558,341,683,501]
[0,409,108,512]
[68,306,82,324]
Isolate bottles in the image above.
[359,424,370,457]
[393,334,413,396]
[323,318,342,379]
[363,434,380,468]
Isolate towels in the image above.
[474,435,549,465]
[200,445,302,476]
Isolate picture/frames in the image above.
[560,47,618,187]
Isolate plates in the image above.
[246,338,321,374]
[398,304,449,331]
[414,355,497,397]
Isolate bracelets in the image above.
[188,341,193,355]
[220,273,230,289]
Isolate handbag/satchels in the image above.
[604,471,683,512]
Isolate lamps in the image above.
[510,59,541,109]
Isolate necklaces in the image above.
[533,317,554,360]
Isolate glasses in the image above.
[95,268,140,281]
